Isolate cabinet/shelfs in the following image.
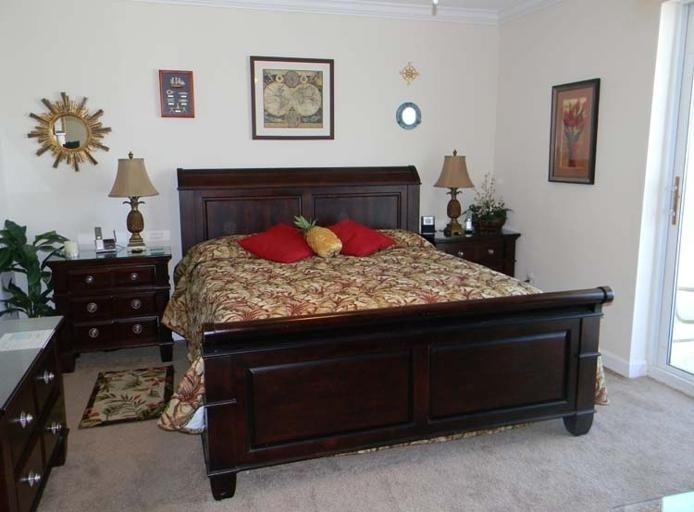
[0,318,72,508]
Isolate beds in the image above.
[177,166,616,503]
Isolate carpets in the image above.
[76,364,175,430]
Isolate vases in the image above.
[469,213,506,237]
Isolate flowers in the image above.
[459,171,514,222]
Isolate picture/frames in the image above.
[250,56,336,141]
[548,78,601,185]
[158,69,194,119]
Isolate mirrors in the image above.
[395,102,422,131]
[27,91,115,173]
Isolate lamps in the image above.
[107,151,161,253]
[432,150,475,236]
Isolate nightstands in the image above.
[46,243,174,375]
[419,225,522,280]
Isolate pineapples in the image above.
[305,227,342,257]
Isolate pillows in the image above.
[235,216,397,264]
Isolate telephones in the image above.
[95,239,116,254]
